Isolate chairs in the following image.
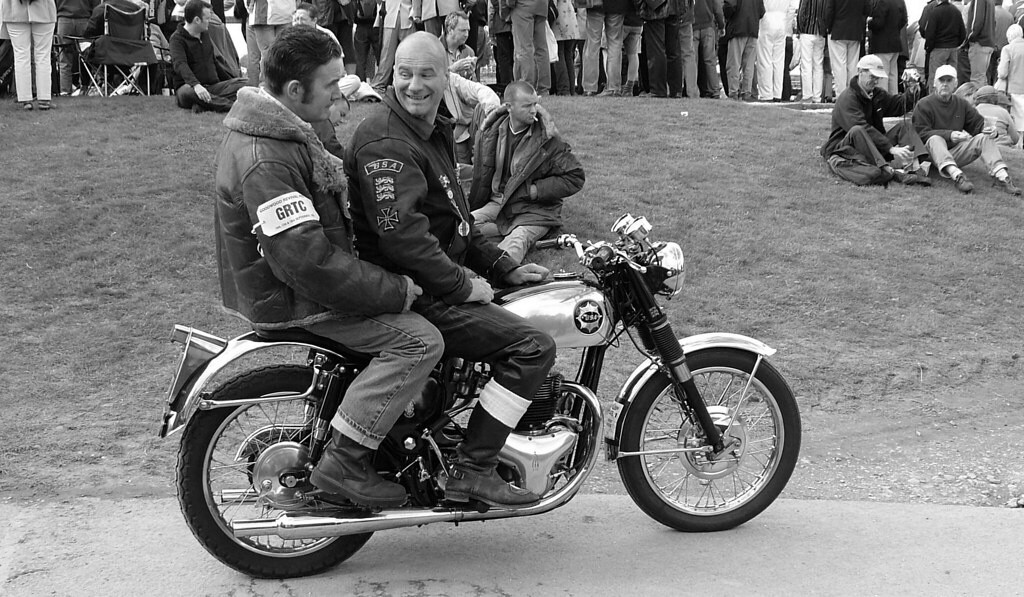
[53,4,172,99]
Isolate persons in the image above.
[468,81,586,266]
[344,31,556,509]
[820,54,932,185]
[0,0,1024,186]
[912,65,1021,194]
[214,24,446,508]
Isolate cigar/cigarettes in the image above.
[533,116,538,121]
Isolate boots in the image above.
[443,403,540,510]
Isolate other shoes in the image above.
[191,103,202,113]
[889,170,918,185]
[912,169,933,185]
[623,81,634,96]
[38,101,56,110]
[952,172,973,192]
[23,102,33,109]
[639,92,667,97]
[596,89,621,96]
[993,175,1022,196]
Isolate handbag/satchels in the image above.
[827,155,892,188]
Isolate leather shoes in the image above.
[310,428,408,507]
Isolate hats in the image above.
[856,55,887,78]
[974,86,997,98]
[996,93,1013,107]
[934,64,957,79]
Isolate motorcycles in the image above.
[157,213,802,579]
